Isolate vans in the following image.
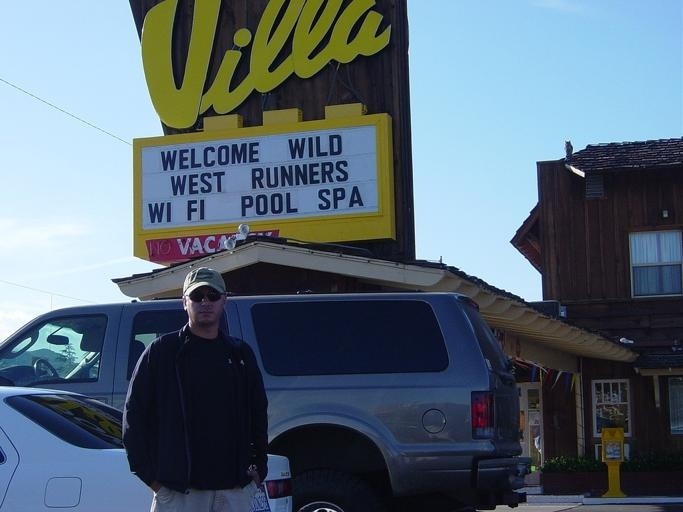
[0,289,533,512]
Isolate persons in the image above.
[122,268,269,511]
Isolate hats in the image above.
[183,267,226,296]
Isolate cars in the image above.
[0,384,294,512]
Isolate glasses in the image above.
[183,292,226,301]
